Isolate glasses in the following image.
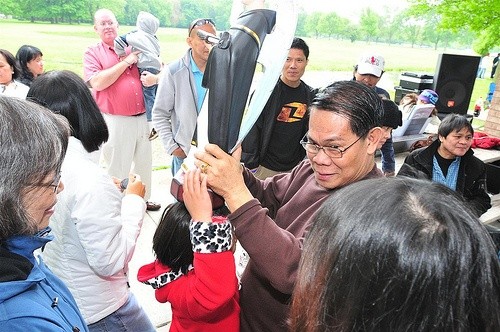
[299,126,370,158]
[23,173,63,195]
[188,18,216,36]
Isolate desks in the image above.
[374,145,500,195]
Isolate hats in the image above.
[416,89,438,105]
[357,51,386,78]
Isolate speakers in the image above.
[432,53,482,116]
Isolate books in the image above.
[400,103,436,136]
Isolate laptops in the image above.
[393,103,434,139]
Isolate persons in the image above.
[0,95,89,332]
[240,37,318,180]
[281,174,500,332]
[0,9,217,212]
[195,80,391,332]
[398,88,442,135]
[26,69,155,332]
[476,52,490,79]
[395,111,492,219]
[490,53,500,78]
[137,167,240,332]
[352,52,403,177]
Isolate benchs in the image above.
[478,192,500,232]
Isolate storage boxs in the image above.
[400,72,433,90]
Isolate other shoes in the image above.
[146,201,161,211]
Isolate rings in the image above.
[200,163,209,173]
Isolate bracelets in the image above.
[122,59,133,66]
